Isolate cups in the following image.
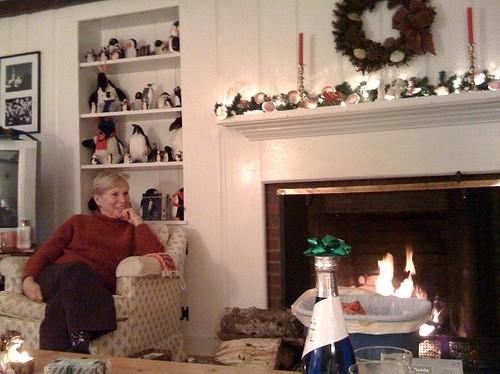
[1,232,16,248]
[352,345,413,374]
[348,361,409,374]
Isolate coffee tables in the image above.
[0,349,304,374]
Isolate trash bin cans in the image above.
[295,295,433,361]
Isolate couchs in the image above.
[0,224,188,361]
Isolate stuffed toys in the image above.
[82,117,124,165]
[88,65,182,113]
[85,48,96,62]
[123,39,137,58]
[98,46,109,60]
[124,124,183,163]
[170,187,184,221]
[108,38,122,59]
[154,21,179,55]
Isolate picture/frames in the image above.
[0,51,41,134]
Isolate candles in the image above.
[299,32,303,64]
[467,7,473,43]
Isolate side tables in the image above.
[0,243,39,256]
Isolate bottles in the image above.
[301,255,360,374]
[16,220,32,249]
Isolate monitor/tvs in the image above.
[0,139,41,245]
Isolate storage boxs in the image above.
[143,193,170,221]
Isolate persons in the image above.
[6,68,24,88]
[5,97,32,126]
[21,172,164,354]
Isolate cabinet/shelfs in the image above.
[79,52,183,170]
